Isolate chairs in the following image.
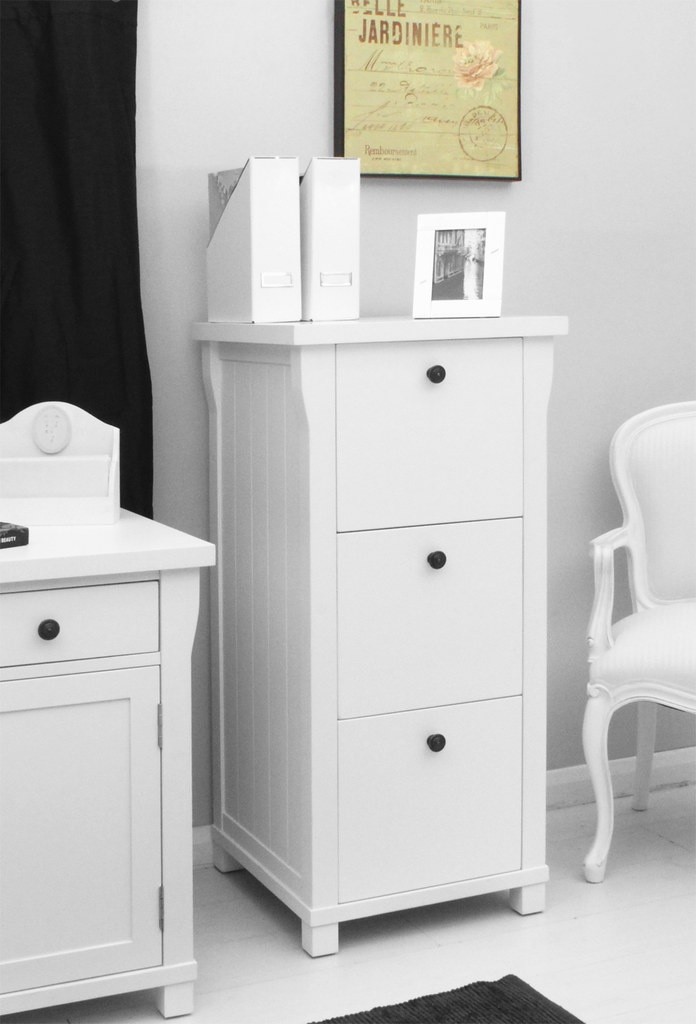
[581,399,695,883]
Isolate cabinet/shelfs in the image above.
[0,400,215,1018]
[191,314,569,958]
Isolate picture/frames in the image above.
[414,212,505,319]
